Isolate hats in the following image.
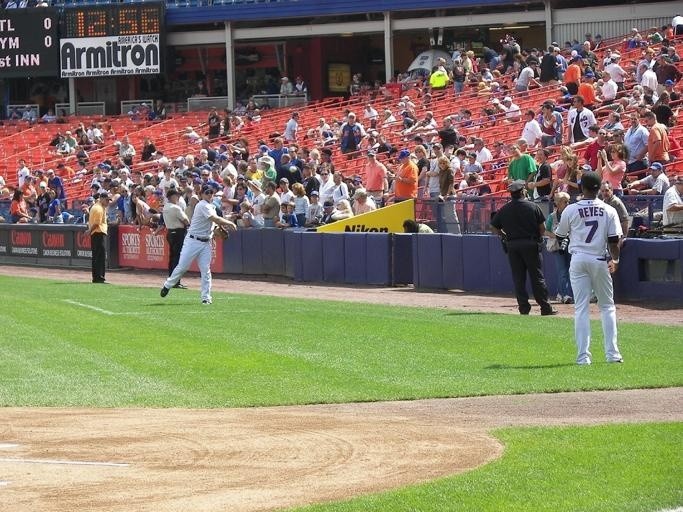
[648,161,663,171]
[141,152,247,183]
[244,176,263,191]
[199,184,213,195]
[579,171,602,190]
[431,137,489,166]
[99,191,112,203]
[366,149,375,159]
[164,189,181,199]
[664,79,674,86]
[506,179,526,194]
[396,149,410,160]
[277,166,364,212]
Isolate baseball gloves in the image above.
[214,223,228,240]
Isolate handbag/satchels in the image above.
[546,238,560,253]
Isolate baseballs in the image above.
[289,166,297,172]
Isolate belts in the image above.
[188,233,213,243]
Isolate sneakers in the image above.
[159,284,169,297]
[605,356,624,364]
[575,355,592,366]
[200,298,211,305]
[554,293,573,304]
[172,282,187,289]
[539,306,558,316]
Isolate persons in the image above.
[84,186,113,283]
[488,180,557,317]
[155,186,236,308]
[548,171,626,363]
[554,176,631,303]
[0,13,683,241]
